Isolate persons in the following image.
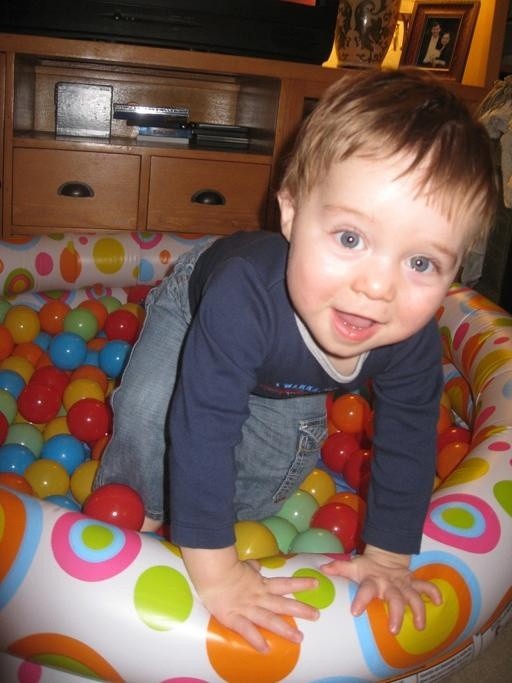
[432,30,454,67]
[419,19,443,64]
[93,64,501,653]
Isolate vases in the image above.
[334,0,402,70]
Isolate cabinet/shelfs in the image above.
[1,33,486,239]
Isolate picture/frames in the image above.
[398,0,481,82]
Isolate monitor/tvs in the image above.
[0,1,342,67]
